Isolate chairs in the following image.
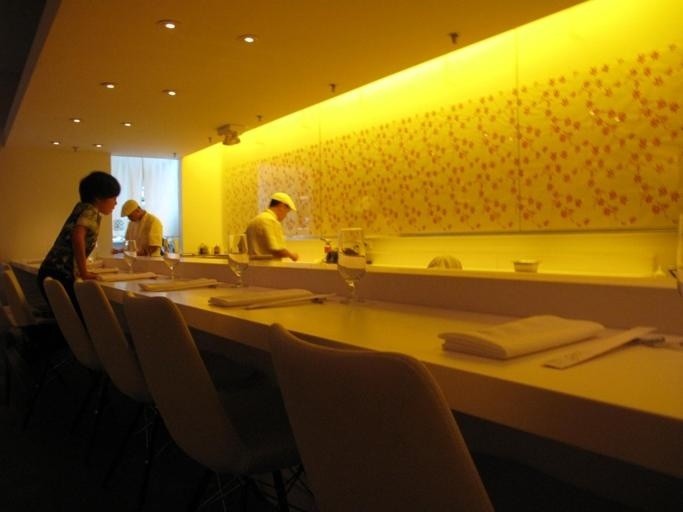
[122,286,308,508]
[1,263,57,380]
[71,279,152,509]
[40,274,112,446]
[267,321,497,510]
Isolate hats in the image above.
[270,192,299,211]
[119,199,140,218]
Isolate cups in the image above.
[513,259,541,273]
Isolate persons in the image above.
[237,192,300,262]
[36,171,121,318]
[119,199,163,257]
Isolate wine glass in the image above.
[227,234,250,289]
[160,236,182,287]
[336,227,368,305]
[120,239,138,279]
